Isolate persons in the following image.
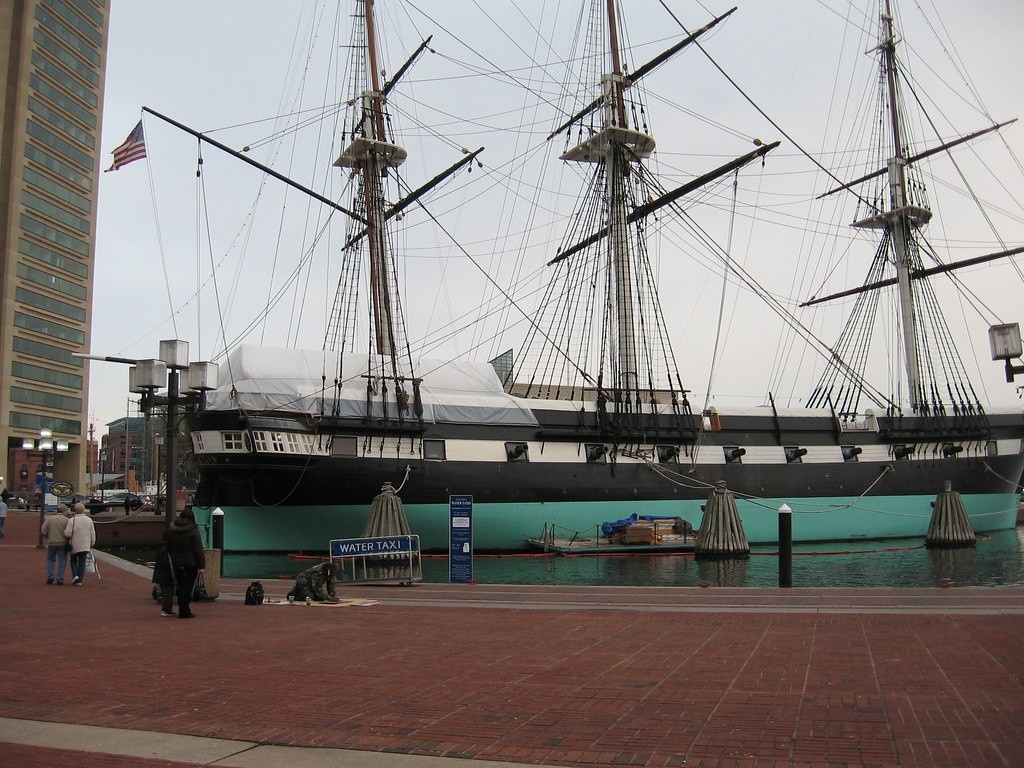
[152,508,206,618]
[287,561,339,602]
[64,502,96,585]
[1,488,9,503]
[41,503,70,584]
[0,496,8,537]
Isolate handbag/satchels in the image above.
[192,571,208,602]
[245,582,265,605]
[84,548,95,572]
[65,543,72,551]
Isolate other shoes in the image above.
[47,578,54,584]
[161,611,176,617]
[71,575,79,585]
[57,581,63,585]
[77,582,82,586]
[1,533,4,538]
[177,614,195,619]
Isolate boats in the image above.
[528,513,699,555]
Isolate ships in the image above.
[185,0,1024,554]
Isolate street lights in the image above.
[23,428,68,549]
[129,340,219,528]
[100,448,107,503]
[154,438,164,515]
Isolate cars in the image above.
[105,493,138,502]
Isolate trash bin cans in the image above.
[192,547,222,602]
